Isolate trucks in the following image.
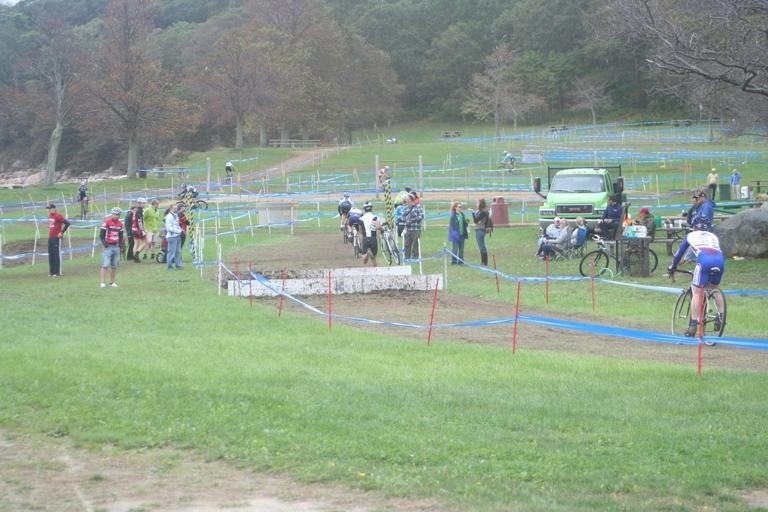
[532,164,628,239]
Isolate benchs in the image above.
[594,225,679,256]
[152,167,189,177]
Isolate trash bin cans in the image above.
[490,196,510,228]
[719,184,730,200]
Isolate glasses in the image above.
[46,204,56,210]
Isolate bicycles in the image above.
[376,176,393,193]
[227,168,236,184]
[668,267,728,346]
[580,234,660,278]
[177,192,209,211]
[80,196,89,222]
[496,161,522,175]
[341,213,400,267]
[155,251,165,263]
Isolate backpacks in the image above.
[486,216,493,234]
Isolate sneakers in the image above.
[685,326,697,338]
[451,253,489,267]
[109,283,118,288]
[715,320,726,331]
[100,282,106,288]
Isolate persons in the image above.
[337,186,425,266]
[99,207,126,288]
[467,199,489,267]
[124,197,191,272]
[378,166,389,188]
[668,223,726,337]
[77,180,90,221]
[224,160,235,177]
[45,204,71,278]
[597,195,622,240]
[179,183,199,199]
[502,150,515,168]
[684,192,716,263]
[706,167,719,201]
[729,169,742,200]
[622,207,656,268]
[536,216,589,261]
[448,202,469,266]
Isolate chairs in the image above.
[536,226,587,260]
[560,178,574,189]
[586,178,601,192]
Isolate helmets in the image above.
[111,207,122,215]
[362,201,374,210]
[137,198,147,203]
[690,215,710,230]
